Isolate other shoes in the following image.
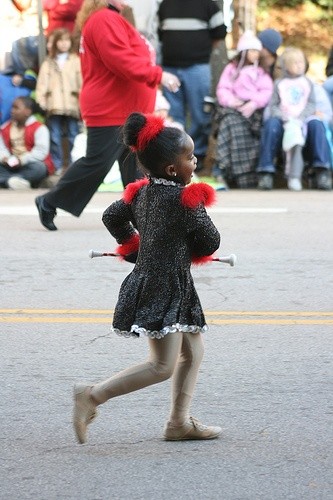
[257,173,272,189]
[287,177,302,191]
[7,176,32,191]
[317,170,333,190]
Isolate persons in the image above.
[263,46,332,191]
[216,32,274,188]
[35,0,181,230]
[74,114,225,444]
[157,0,228,171]
[258,28,333,191]
[324,49,333,95]
[0,95,50,190]
[35,28,84,173]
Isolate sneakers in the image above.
[162,416,223,439]
[73,379,98,444]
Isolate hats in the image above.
[236,26,262,51]
[257,28,282,53]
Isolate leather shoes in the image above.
[35,194,57,230]
